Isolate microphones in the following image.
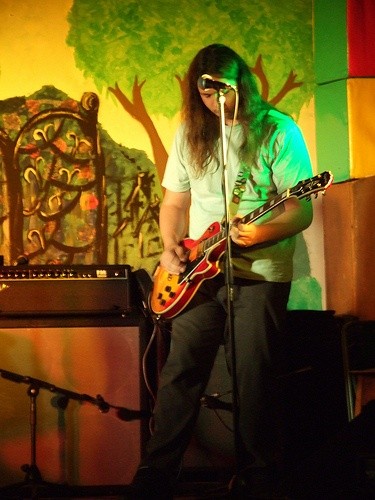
[199,74,237,92]
[96,394,109,413]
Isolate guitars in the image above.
[152,170,334,319]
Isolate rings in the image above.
[244,241,248,248]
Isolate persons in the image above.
[128,44,314,500]
